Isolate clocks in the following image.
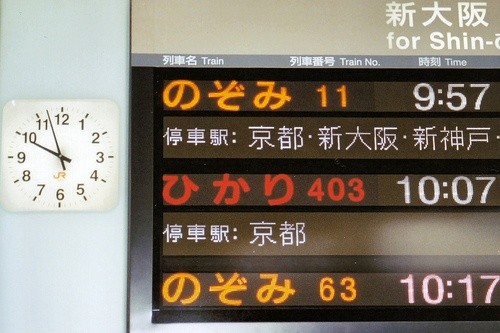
[1,97,121,212]
